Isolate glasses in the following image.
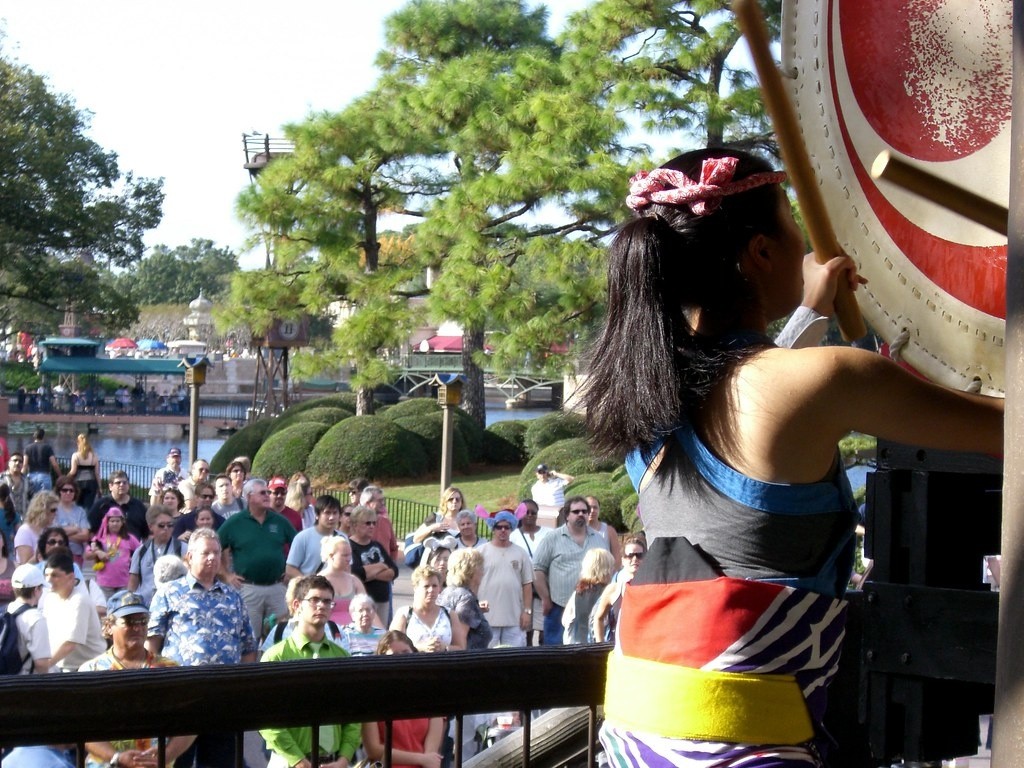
[449,497,461,503]
[536,470,547,474]
[115,618,149,628]
[343,512,352,516]
[272,490,287,496]
[525,510,538,516]
[363,520,376,526]
[623,552,644,560]
[298,596,336,611]
[568,508,590,515]
[10,459,394,545]
[252,490,273,495]
[347,490,357,495]
[494,525,511,531]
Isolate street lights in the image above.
[427,373,470,503]
[177,357,214,469]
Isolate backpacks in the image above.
[0,603,34,676]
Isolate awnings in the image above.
[413,335,570,352]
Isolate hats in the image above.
[10,564,45,589]
[536,464,549,472]
[107,590,151,620]
[268,477,288,490]
[475,502,528,531]
[169,448,182,459]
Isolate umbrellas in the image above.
[109,337,167,350]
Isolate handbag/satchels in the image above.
[404,533,425,570]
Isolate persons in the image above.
[107,348,169,360]
[0,428,647,768]
[585,148,1006,768]
[18,383,186,416]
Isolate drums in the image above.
[774,0,1013,411]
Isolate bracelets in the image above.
[110,752,119,768]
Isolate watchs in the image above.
[524,608,531,614]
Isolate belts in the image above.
[317,752,339,764]
